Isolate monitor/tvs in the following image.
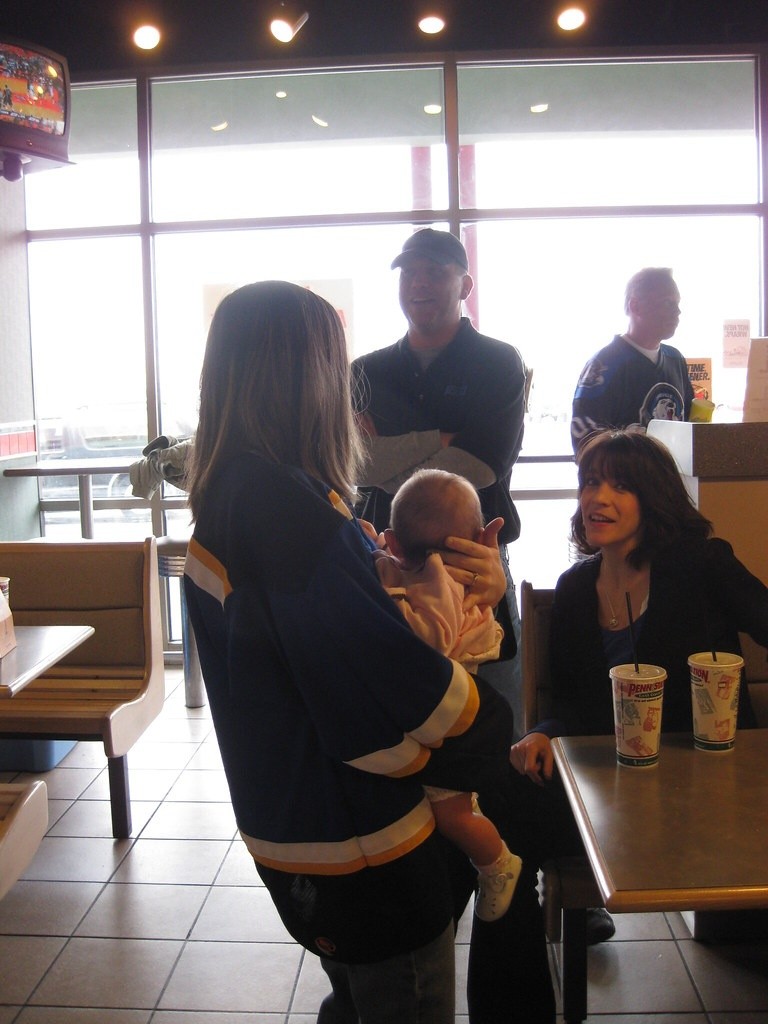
[0,33,71,160]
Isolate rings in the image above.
[471,572,479,587]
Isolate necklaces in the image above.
[598,569,631,628]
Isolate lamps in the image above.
[268,0,309,43]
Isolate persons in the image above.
[359,468,522,921]
[571,268,696,466]
[508,423,768,945]
[350,227,525,734]
[181,280,514,1024]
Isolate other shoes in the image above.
[473,854,523,922]
[587,908,615,944]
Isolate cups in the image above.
[0,577,10,606]
[609,664,667,769]
[687,652,745,753]
[689,397,715,423]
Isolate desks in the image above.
[544,727,768,1024]
[0,625,94,698]
[2,459,184,539]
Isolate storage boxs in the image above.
[0,739,79,772]
[0,589,16,659]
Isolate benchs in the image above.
[0,538,166,838]
[0,780,50,903]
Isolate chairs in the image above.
[519,581,768,942]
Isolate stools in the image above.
[156,542,202,707]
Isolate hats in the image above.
[391,227,468,270]
